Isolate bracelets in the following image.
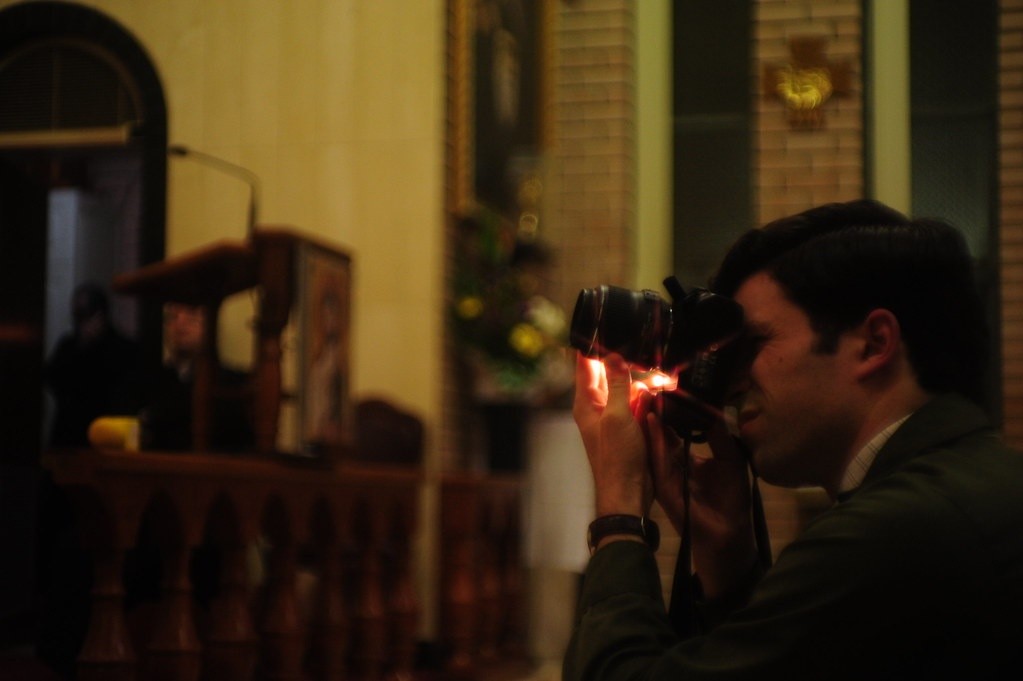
[587,514,660,557]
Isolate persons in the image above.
[561,198,1023,681]
[46,280,255,605]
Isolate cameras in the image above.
[569,274,750,441]
